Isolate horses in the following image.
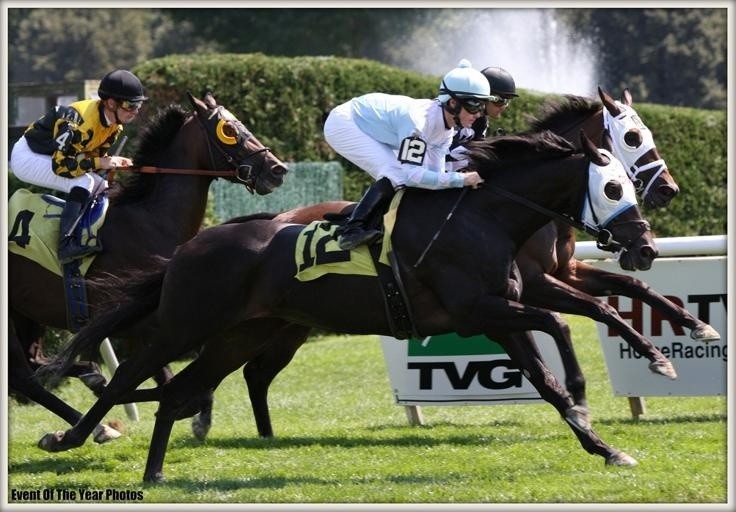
[39,129,659,488]
[191,86,722,441]
[9,91,288,444]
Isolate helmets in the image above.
[437,59,495,103]
[481,65,521,98]
[99,68,149,103]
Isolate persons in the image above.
[323,60,497,247]
[11,70,143,263]
[445,66,520,169]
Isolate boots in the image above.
[56,186,100,264]
[338,176,396,251]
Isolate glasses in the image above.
[491,96,512,106]
[463,100,487,114]
